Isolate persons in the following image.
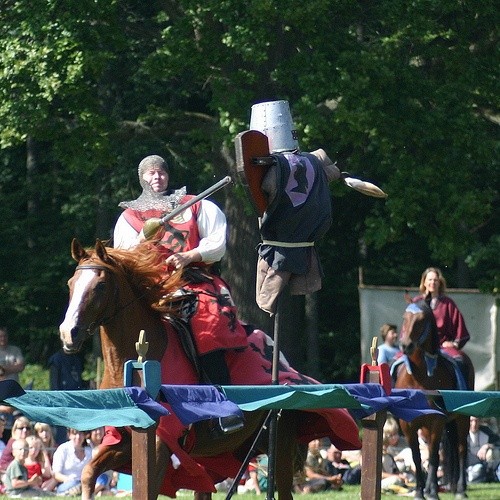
[0,323,500,500]
[391,268,470,438]
[112,155,248,433]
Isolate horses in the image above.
[58,238,309,500]
[391,289,474,500]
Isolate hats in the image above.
[381,323,396,338]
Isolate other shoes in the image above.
[211,412,244,437]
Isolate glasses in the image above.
[17,427,31,431]
